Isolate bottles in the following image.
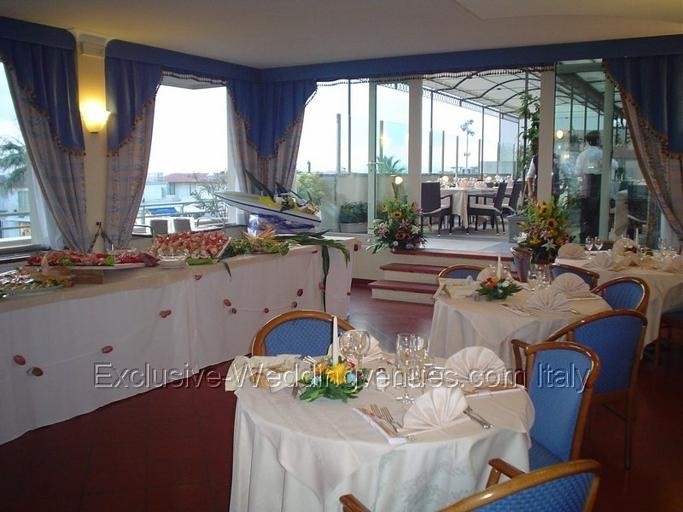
[474,290,480,302]
[526,269,551,292]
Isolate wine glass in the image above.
[438,174,512,189]
[337,328,447,406]
[585,235,683,277]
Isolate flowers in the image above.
[362,197,430,257]
[511,191,571,264]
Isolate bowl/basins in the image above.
[156,247,190,269]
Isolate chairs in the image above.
[0,216,682,510]
[412,165,650,249]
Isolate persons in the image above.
[526,153,570,210]
[574,130,618,244]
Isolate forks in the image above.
[356,404,402,438]
[466,407,494,429]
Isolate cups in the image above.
[489,263,514,281]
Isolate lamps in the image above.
[78,109,113,135]
